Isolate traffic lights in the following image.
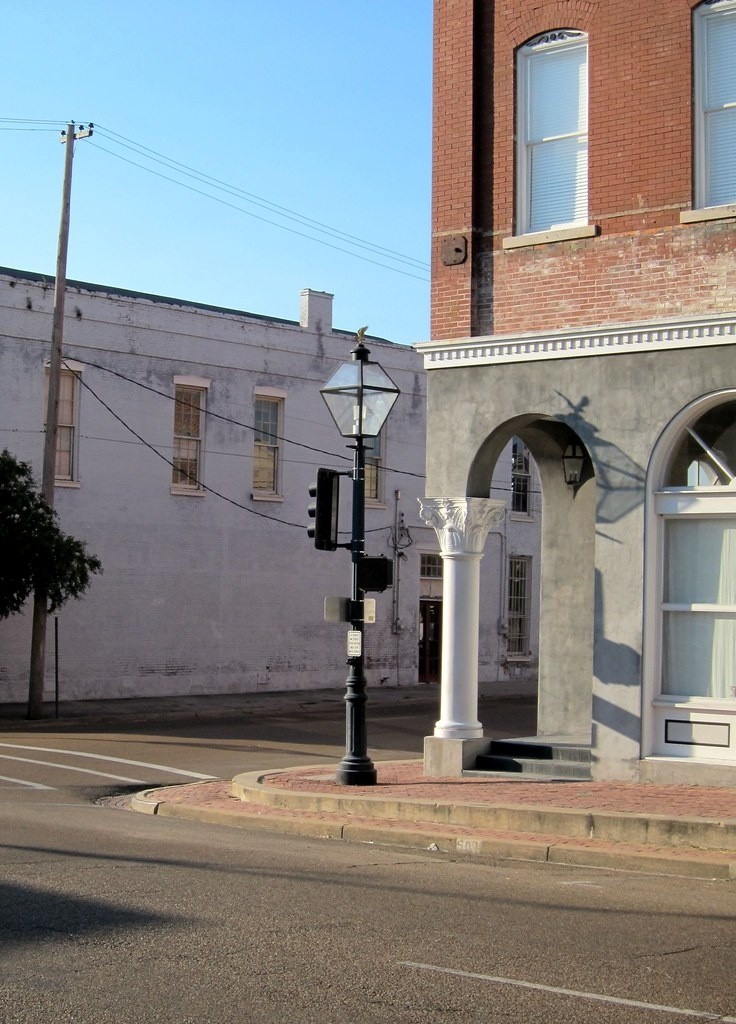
[307,467,339,552]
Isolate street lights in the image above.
[318,325,401,787]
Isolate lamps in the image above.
[561,422,585,485]
